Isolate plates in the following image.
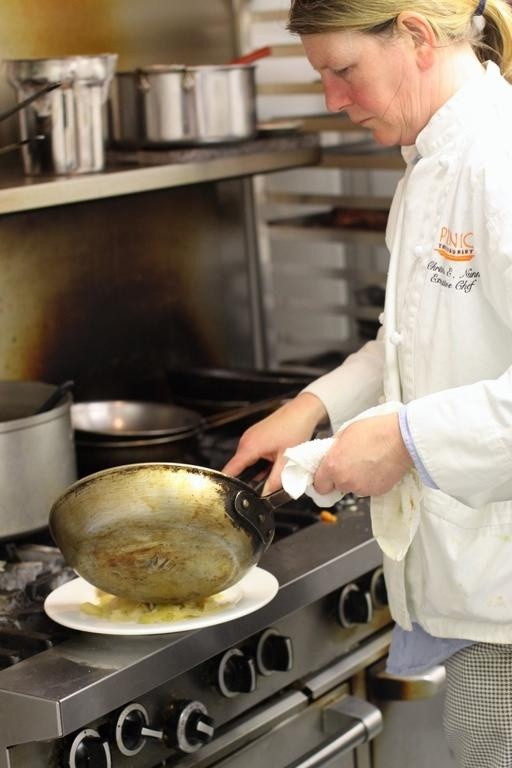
[42,565,278,642]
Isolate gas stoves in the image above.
[0,480,390,766]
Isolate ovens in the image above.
[202,640,386,765]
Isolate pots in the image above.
[1,383,83,539]
[52,464,330,605]
[76,395,301,466]
[106,61,253,145]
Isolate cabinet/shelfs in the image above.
[230,1,407,375]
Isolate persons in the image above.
[222,2,512,767]
